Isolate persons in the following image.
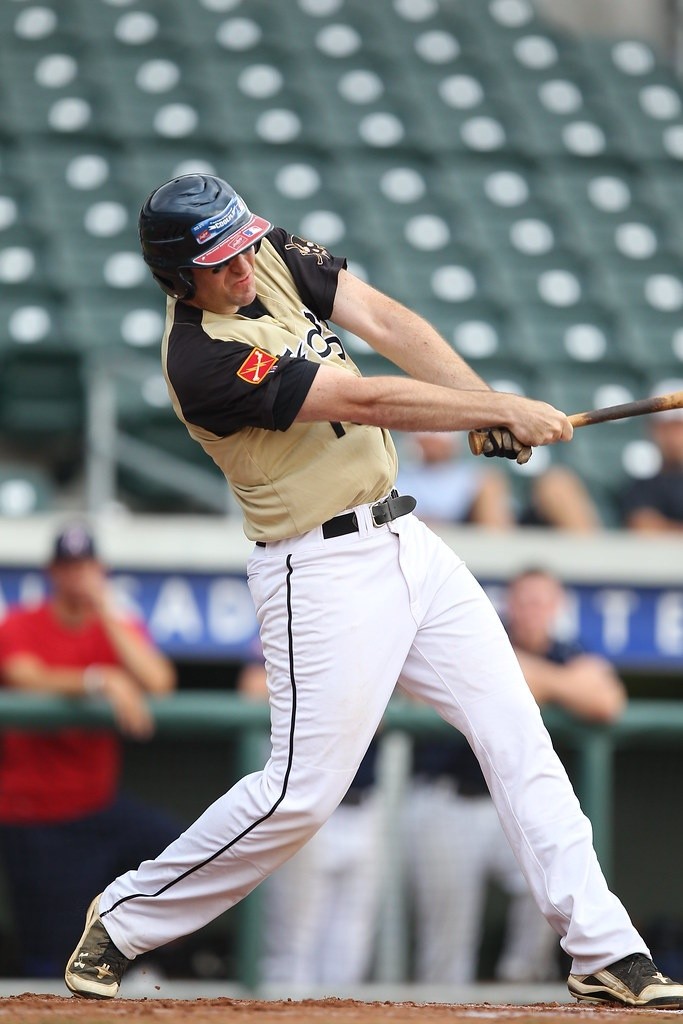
[610,410,683,531]
[392,429,516,530]
[236,619,411,989]
[407,566,628,985]
[66,172,683,1010]
[0,523,223,982]
[515,463,608,532]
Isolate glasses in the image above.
[211,242,252,275]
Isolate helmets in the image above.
[138,173,275,300]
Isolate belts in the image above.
[254,491,416,548]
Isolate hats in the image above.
[53,527,97,565]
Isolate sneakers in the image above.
[63,891,131,999]
[566,953,683,1009]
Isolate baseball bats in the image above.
[467,389,683,457]
